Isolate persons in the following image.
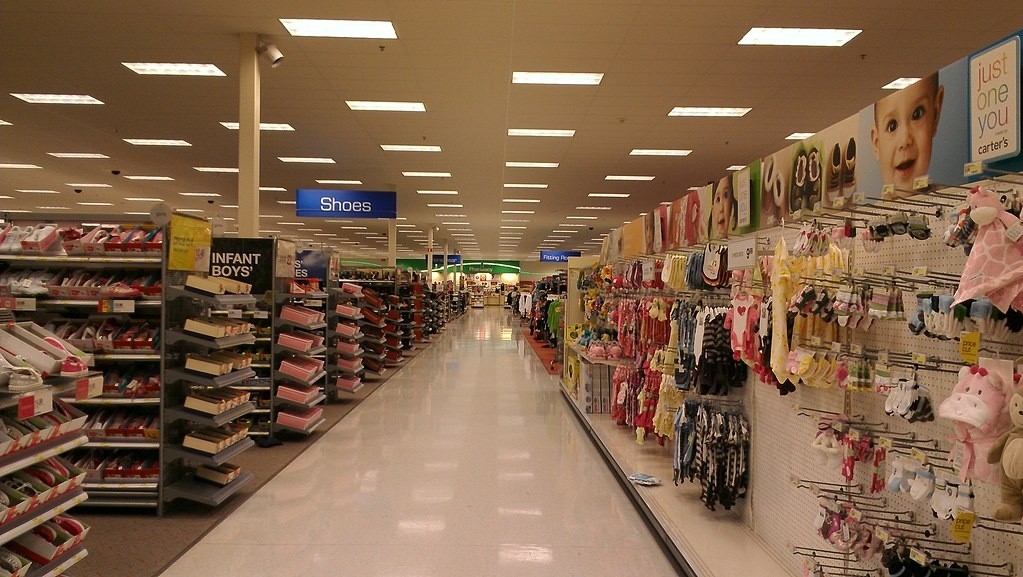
[644,211,657,255]
[707,173,738,240]
[870,69,947,206]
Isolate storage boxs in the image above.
[43,318,160,349]
[103,370,126,398]
[0,512,90,577]
[336,283,400,388]
[197,463,241,487]
[21,224,59,255]
[61,223,163,257]
[276,305,325,429]
[0,455,87,525]
[0,396,88,457]
[184,316,251,342]
[183,389,251,418]
[0,224,12,243]
[82,409,160,442]
[0,321,85,386]
[184,350,252,379]
[183,424,249,456]
[74,453,160,483]
[567,355,617,414]
[0,267,161,299]
[186,276,252,297]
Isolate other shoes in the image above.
[761,136,858,226]
[0,225,162,577]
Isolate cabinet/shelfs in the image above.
[0,212,256,519]
[0,308,102,577]
[357,292,387,381]
[400,267,484,349]
[295,250,365,405]
[210,237,329,448]
[339,267,404,365]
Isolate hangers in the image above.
[676,290,732,308]
[682,394,743,416]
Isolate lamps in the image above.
[255,35,283,68]
